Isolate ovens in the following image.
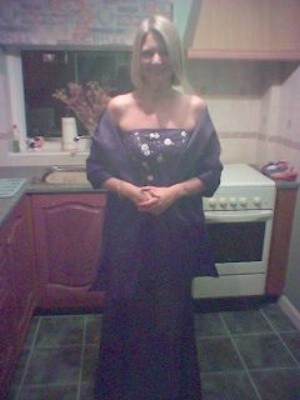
[191,186,276,299]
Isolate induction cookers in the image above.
[219,161,276,186]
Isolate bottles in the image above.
[12,125,20,152]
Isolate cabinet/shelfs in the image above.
[0,194,106,400]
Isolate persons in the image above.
[86,15,223,400]
